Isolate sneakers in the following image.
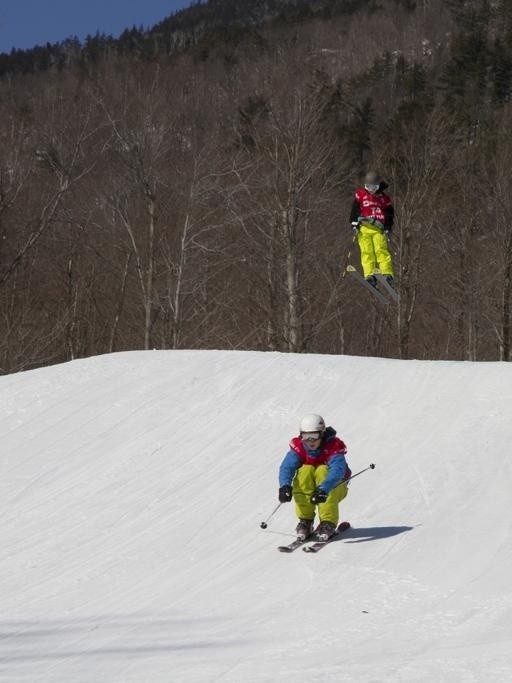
[318,520,336,541]
[366,275,377,288]
[296,519,312,538]
[383,273,394,288]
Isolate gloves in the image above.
[310,492,327,504]
[279,485,293,502]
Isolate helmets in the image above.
[365,170,378,183]
[301,413,325,432]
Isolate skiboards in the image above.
[346,265,402,304]
[278,522,350,553]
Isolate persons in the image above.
[279,412,350,541]
[349,170,396,285]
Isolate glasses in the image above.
[301,432,321,440]
[364,184,379,191]
